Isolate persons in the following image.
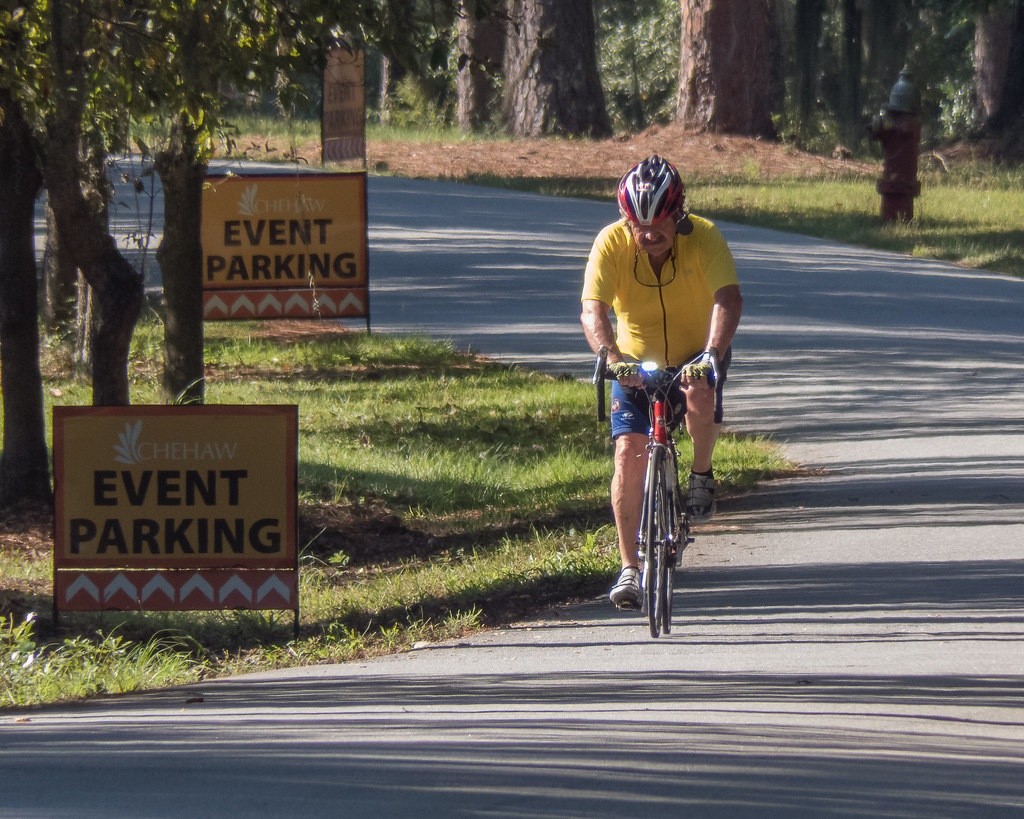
[581,155,744,607]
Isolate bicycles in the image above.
[591,345,721,637]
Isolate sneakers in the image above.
[685,471,717,523]
[608,567,644,611]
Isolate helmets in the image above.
[617,153,686,226]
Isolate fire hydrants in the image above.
[865,66,924,225]
